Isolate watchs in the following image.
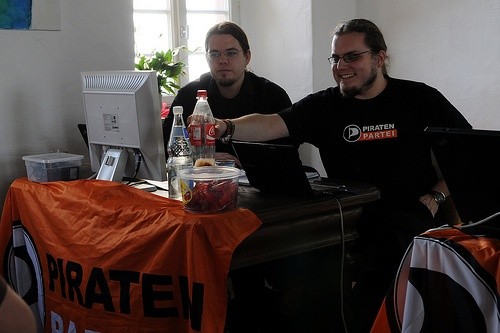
[432,190,446,204]
[220,119,236,147]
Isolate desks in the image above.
[9,182,380,333]
[370,225,500,333]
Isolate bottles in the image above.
[190,89,216,167]
[166,106,193,202]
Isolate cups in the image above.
[217,160,236,168]
[166,164,191,200]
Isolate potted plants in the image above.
[133,50,184,109]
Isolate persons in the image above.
[0,274,37,333]
[190,17,474,283]
[161,22,294,188]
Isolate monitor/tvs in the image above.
[79,70,167,193]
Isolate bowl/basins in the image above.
[179,167,241,215]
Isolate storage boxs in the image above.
[23,153,84,182]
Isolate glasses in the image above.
[206,49,244,60]
[328,50,373,65]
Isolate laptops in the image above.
[231,140,347,200]
